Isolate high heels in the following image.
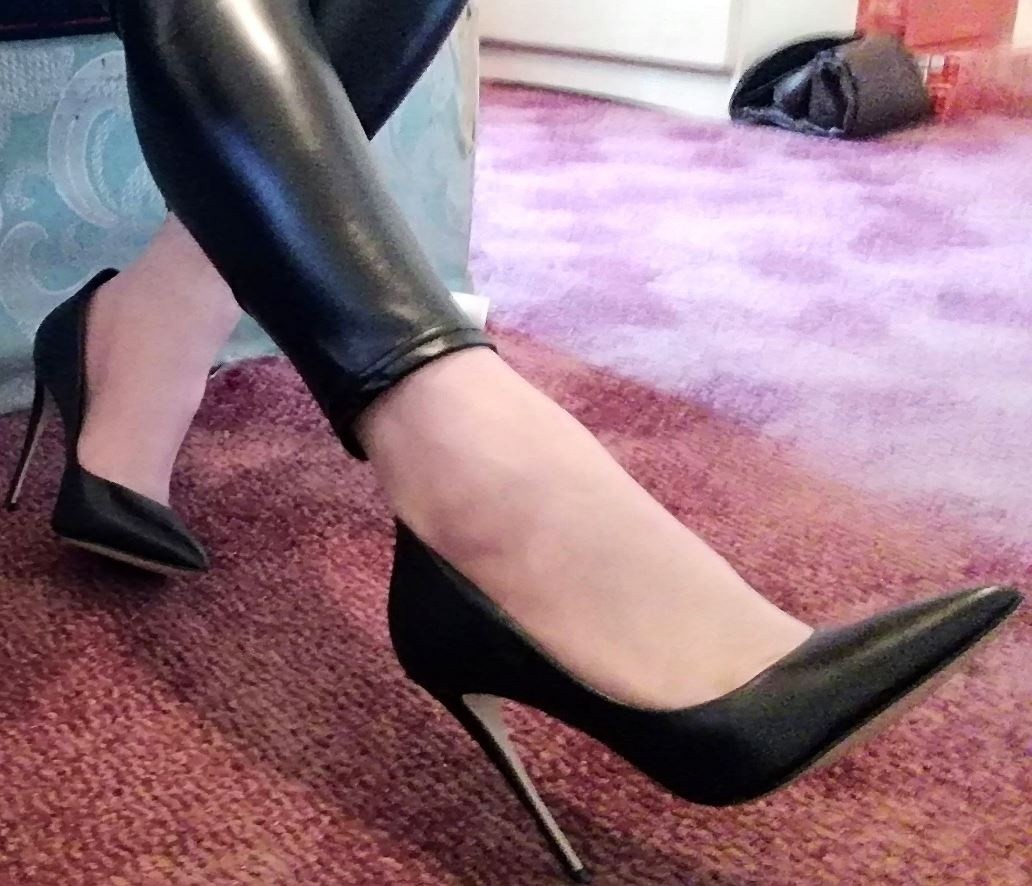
[4,267,210,578]
[387,513,1024,884]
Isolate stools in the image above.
[0,5,479,419]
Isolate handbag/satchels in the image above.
[728,31,934,139]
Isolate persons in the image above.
[0,0,1024,883]
